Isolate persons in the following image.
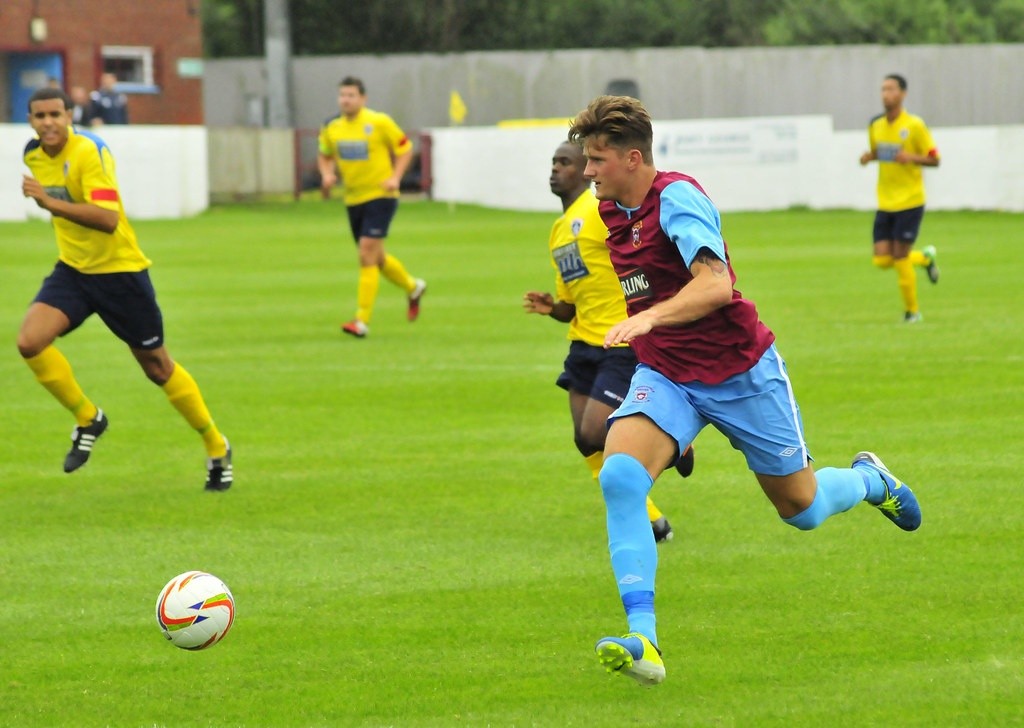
[567,95,922,683]
[88,72,128,125]
[522,136,693,545]
[16,89,233,491]
[318,77,428,337]
[66,87,89,126]
[861,74,939,321]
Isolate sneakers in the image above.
[406,279,427,321]
[594,632,666,685]
[342,321,366,337]
[64,407,108,473]
[904,310,923,323]
[922,245,939,283]
[851,451,921,531]
[204,437,233,492]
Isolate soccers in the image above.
[153,569,236,651]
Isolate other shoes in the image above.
[650,516,673,544]
[675,444,694,477]
